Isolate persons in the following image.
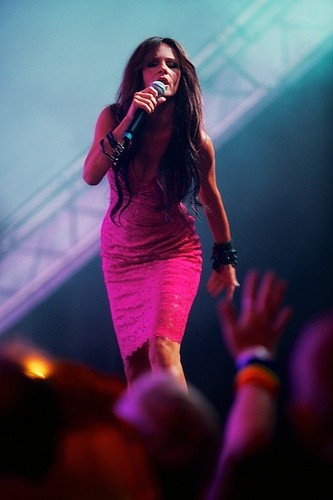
[80,36,240,396]
[0,265,333,500]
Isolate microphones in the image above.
[123,80,166,142]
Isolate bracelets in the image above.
[99,131,118,159]
[210,240,240,272]
